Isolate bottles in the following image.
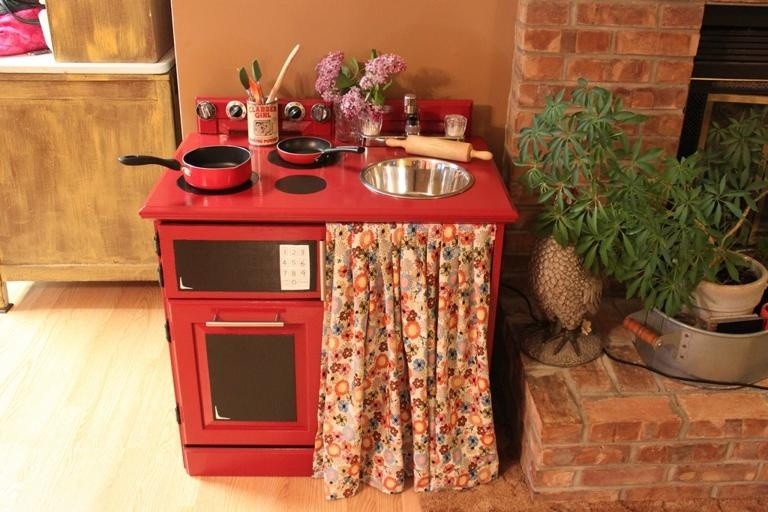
[404,93,420,136]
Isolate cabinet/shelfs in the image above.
[43,1,173,64]
[151,220,324,479]
[0,73,180,313]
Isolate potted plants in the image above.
[511,75,768,332]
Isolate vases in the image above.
[354,117,384,139]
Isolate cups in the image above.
[247,98,279,147]
[444,114,467,140]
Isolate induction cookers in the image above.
[176,96,341,195]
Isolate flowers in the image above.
[311,47,408,124]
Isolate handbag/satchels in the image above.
[0,7,48,55]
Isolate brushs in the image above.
[239,60,266,104]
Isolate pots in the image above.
[276,136,366,165]
[623,304,768,390]
[118,144,252,191]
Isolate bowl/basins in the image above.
[359,156,475,200]
